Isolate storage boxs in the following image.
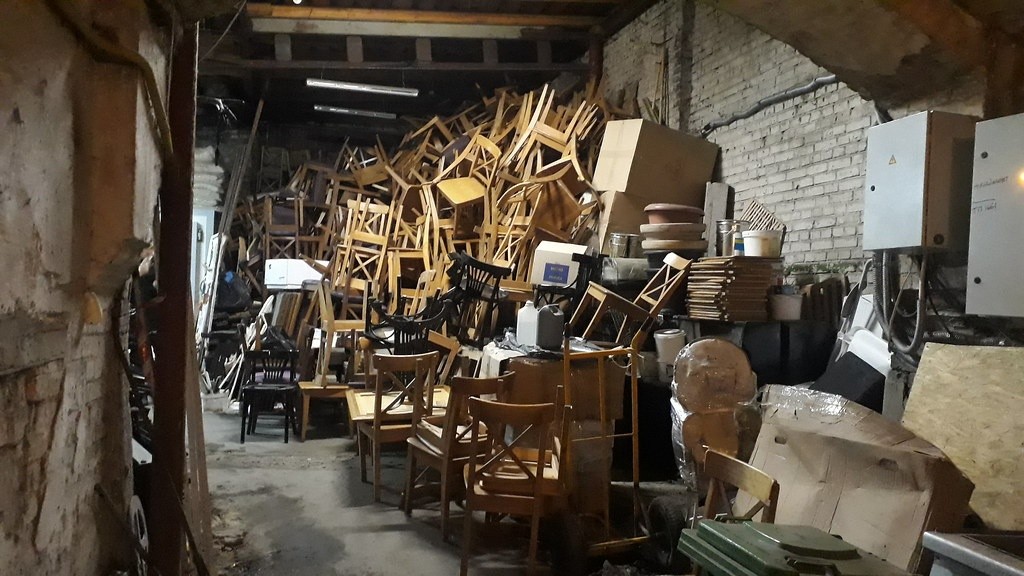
[590,117,721,208]
[478,333,629,424]
[577,189,655,259]
[505,420,617,526]
[728,382,978,575]
[530,240,598,292]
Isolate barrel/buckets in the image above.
[536,304,564,352]
[742,229,781,260]
[517,300,538,347]
[715,219,751,258]
[770,294,802,321]
[609,232,640,259]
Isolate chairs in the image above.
[205,73,782,576]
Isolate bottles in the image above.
[733,224,744,257]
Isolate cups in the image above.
[773,284,794,296]
[638,351,658,382]
[654,329,687,384]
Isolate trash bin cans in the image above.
[676,519,916,576]
